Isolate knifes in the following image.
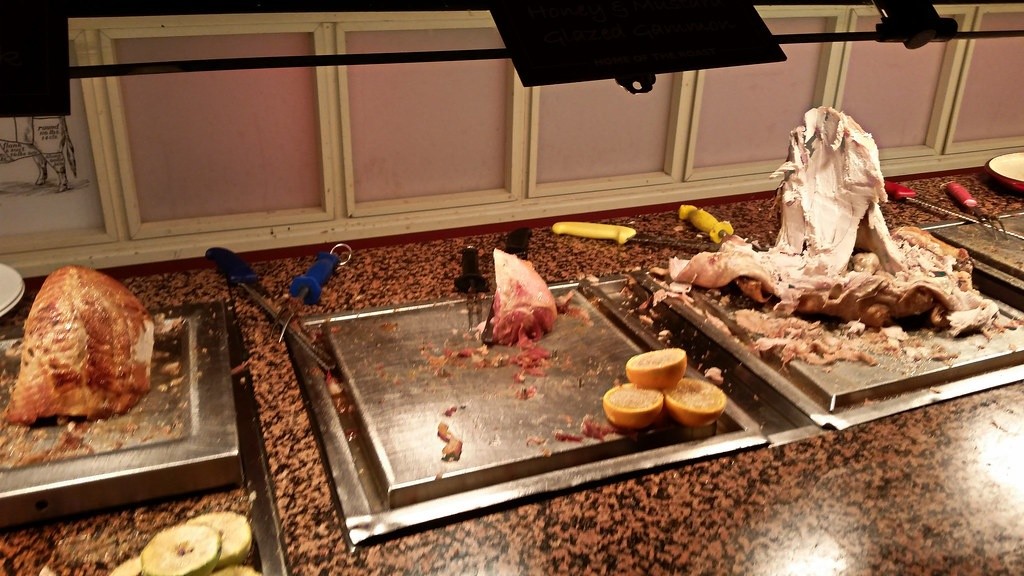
[552,222,720,250]
[482,227,530,344]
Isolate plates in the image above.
[985,152,1024,192]
[0,263,25,317]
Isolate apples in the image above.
[106,507,262,576]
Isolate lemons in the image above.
[601,348,728,431]
